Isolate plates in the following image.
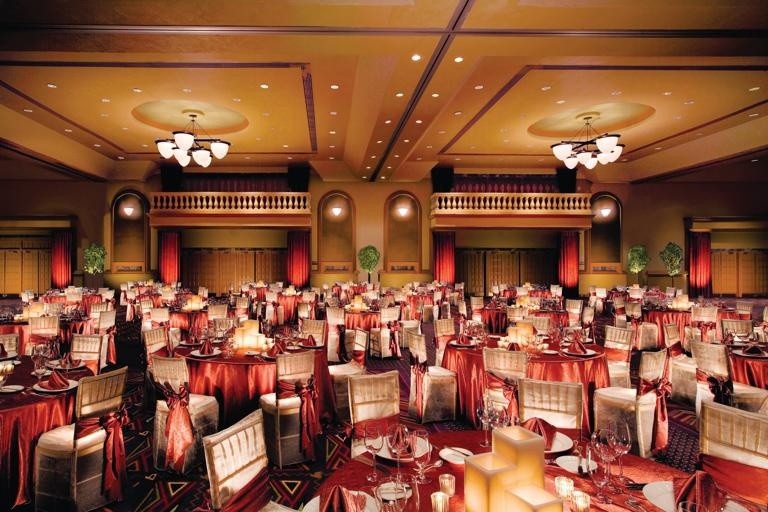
[302,491,381,511]
[722,327,768,354]
[451,331,598,357]
[641,477,747,512]
[542,430,598,475]
[440,445,474,467]
[2,288,324,396]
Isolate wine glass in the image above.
[477,398,520,448]
[361,422,435,487]
[548,319,584,349]
[325,285,737,313]
[461,318,485,343]
[584,417,638,504]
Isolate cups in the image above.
[376,474,456,511]
[556,474,590,510]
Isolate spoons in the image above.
[423,458,443,475]
